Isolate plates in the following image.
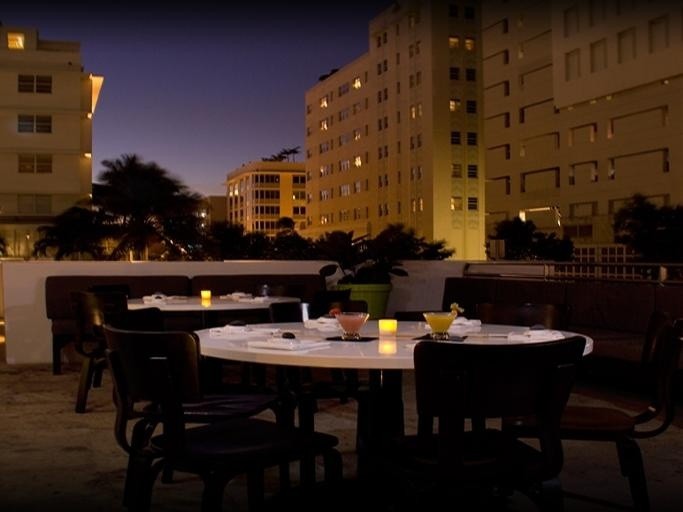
[247,338,332,355]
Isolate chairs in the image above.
[46,274,680,510]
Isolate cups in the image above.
[335,312,370,341]
[422,312,456,340]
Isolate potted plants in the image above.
[319,225,408,321]
